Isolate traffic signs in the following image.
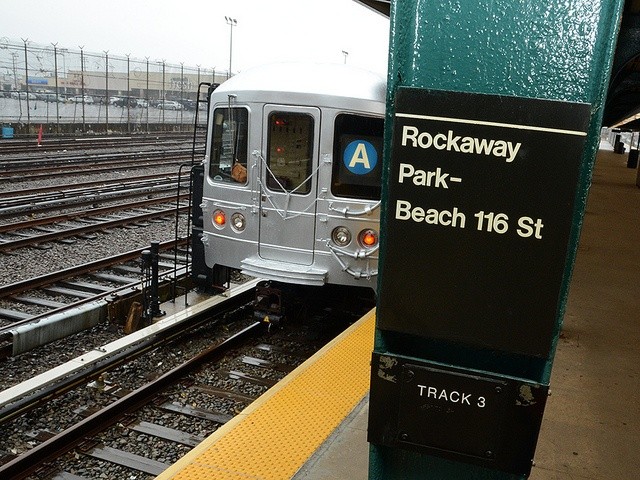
[375,82,593,355]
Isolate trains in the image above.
[207,66,386,308]
